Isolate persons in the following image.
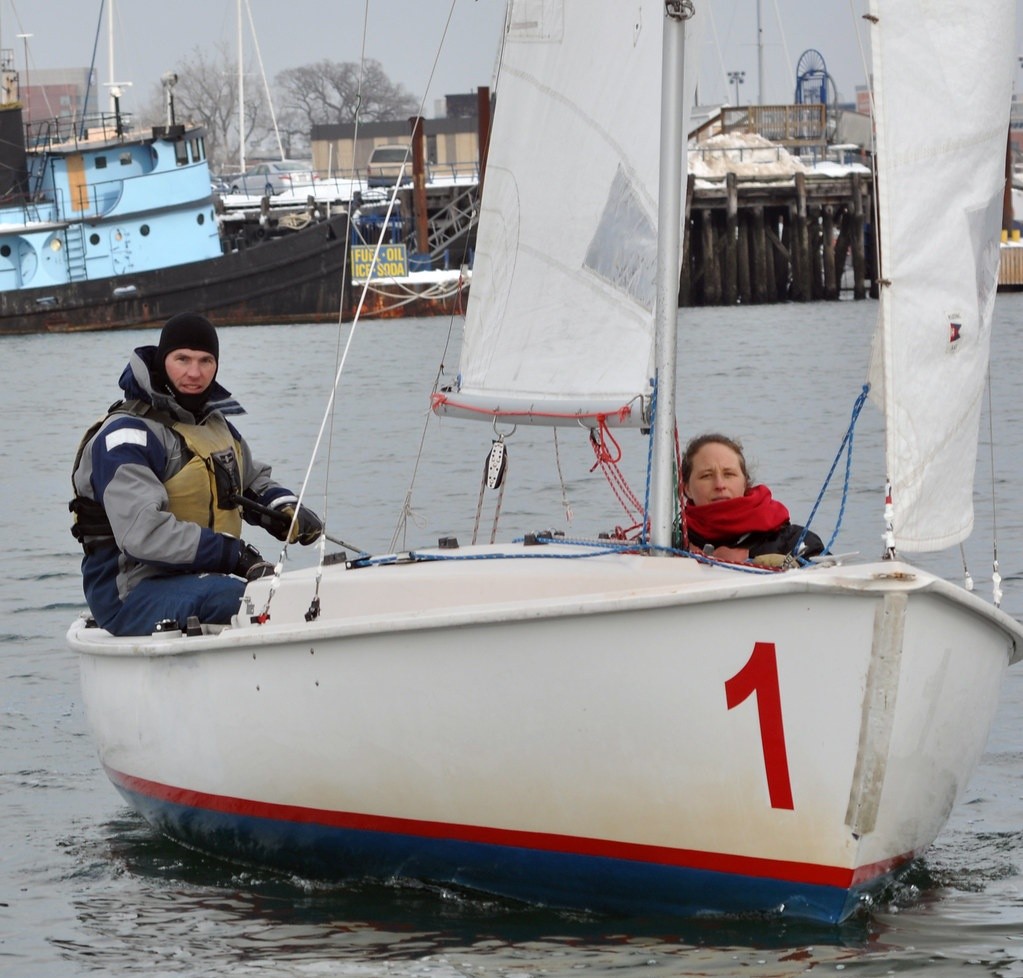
[673,432,835,572]
[67,314,324,636]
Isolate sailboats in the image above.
[66,0,1023,929]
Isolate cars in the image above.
[230,161,321,197]
[367,144,413,190]
[208,168,230,194]
[1013,163,1023,188]
[827,144,858,161]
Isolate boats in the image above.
[1,0,354,335]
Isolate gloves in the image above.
[238,538,274,580]
[270,501,321,546]
